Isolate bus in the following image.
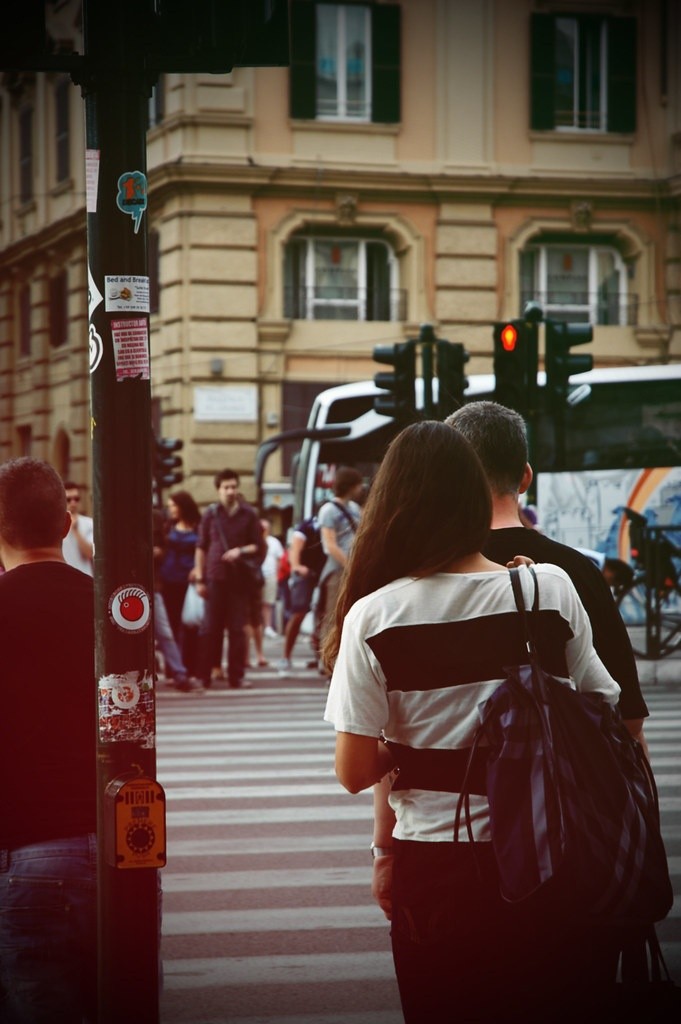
[253,364,681,636]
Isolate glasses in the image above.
[66,496,81,502]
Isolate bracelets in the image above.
[372,846,394,858]
[194,578,205,583]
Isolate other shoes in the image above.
[278,657,294,679]
[229,679,252,689]
[212,669,224,677]
[175,677,204,691]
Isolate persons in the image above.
[372,401,649,921]
[277,499,331,671]
[572,548,636,587]
[260,514,285,638]
[319,420,624,1023]
[152,490,203,690]
[61,481,94,579]
[188,469,269,693]
[1,456,97,1023]
[309,467,364,675]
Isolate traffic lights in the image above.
[371,339,416,428]
[439,338,470,420]
[160,438,183,488]
[491,319,534,419]
[546,318,594,419]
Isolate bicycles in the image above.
[615,507,681,659]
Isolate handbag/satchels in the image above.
[473,566,674,926]
[606,924,681,1024]
[244,557,265,586]
[180,583,206,626]
